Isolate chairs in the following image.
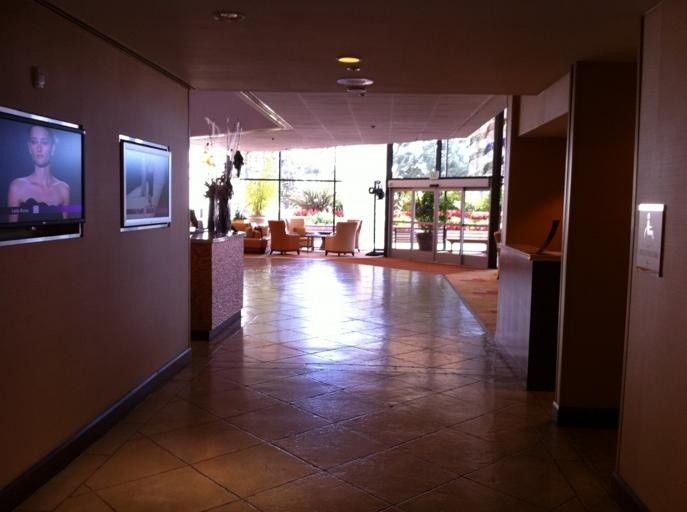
[494,230,501,280]
[344,220,363,255]
[231,222,267,253]
[324,222,357,257]
[286,218,314,252]
[268,221,300,255]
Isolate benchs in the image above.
[447,239,489,253]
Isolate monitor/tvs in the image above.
[120,139,172,228]
[0,111,86,242]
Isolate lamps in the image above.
[365,180,384,256]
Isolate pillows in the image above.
[245,226,265,239]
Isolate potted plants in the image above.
[245,180,271,225]
[403,191,459,251]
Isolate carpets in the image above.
[326,257,481,275]
[447,268,499,335]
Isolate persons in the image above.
[145,155,154,209]
[8,124,71,223]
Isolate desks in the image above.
[304,235,328,253]
